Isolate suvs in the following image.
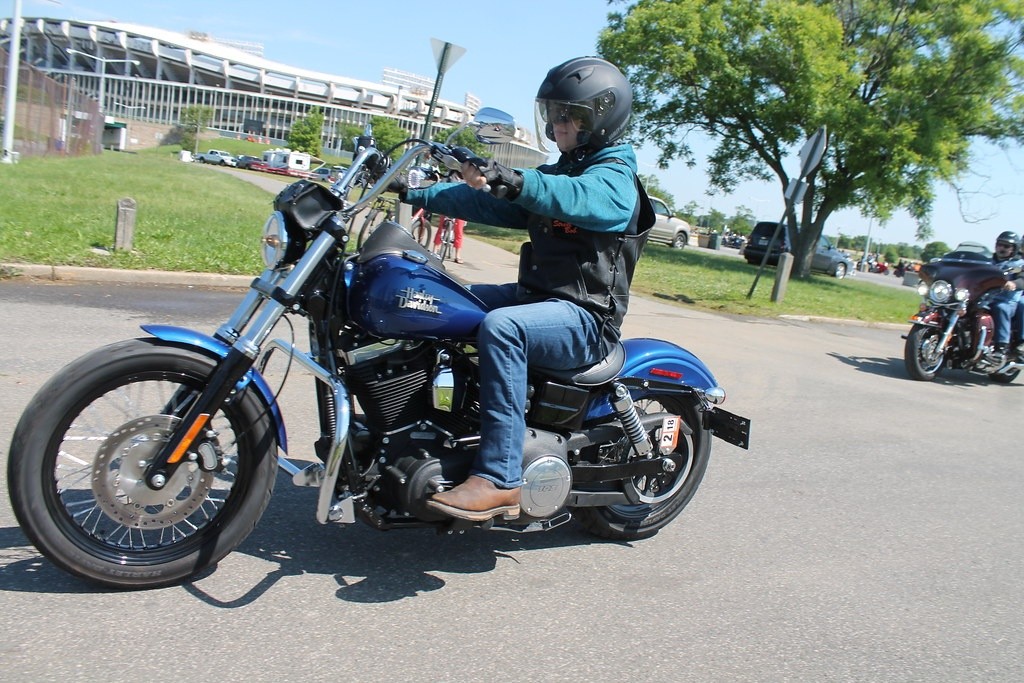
[743,221,854,279]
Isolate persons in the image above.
[355,57,656,523]
[870,251,914,277]
[901,231,1024,352]
[433,215,465,264]
[712,228,744,249]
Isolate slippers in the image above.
[455,256,461,262]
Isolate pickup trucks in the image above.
[197,147,237,167]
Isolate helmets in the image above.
[998,231,1020,256]
[533,56,632,153]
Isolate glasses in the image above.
[997,243,1015,248]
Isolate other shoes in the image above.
[990,345,1006,361]
[1016,342,1024,354]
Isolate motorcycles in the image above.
[6,104,752,588]
[903,240,1023,382]
[722,232,916,277]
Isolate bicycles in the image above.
[357,195,456,264]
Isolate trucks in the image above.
[262,148,312,175]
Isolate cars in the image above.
[310,168,338,182]
[237,156,262,170]
[647,195,692,250]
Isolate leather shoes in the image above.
[426,475,522,521]
